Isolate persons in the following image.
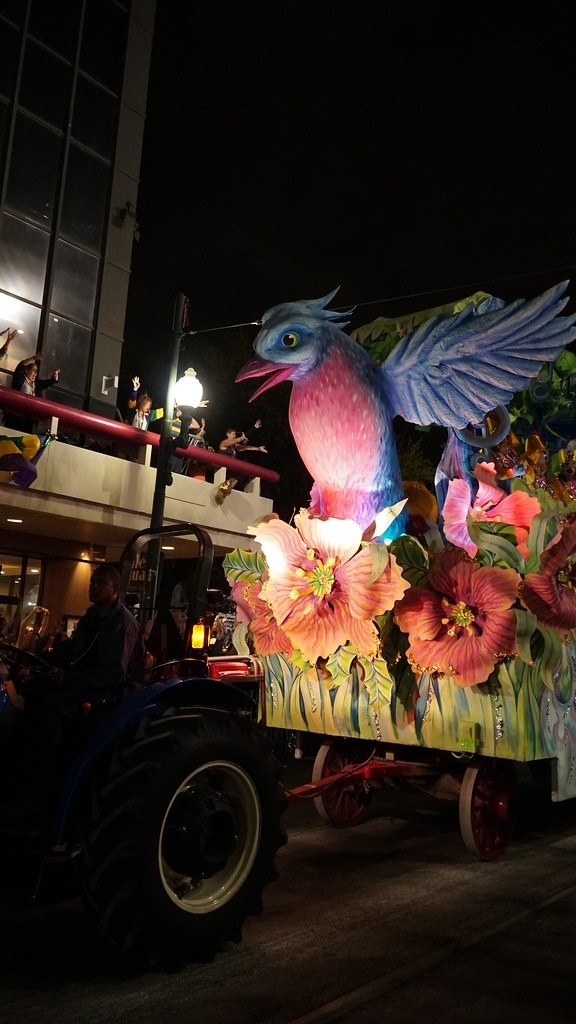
[22,567,148,767]
[172,406,268,492]
[0,328,61,434]
[116,376,177,462]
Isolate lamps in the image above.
[101,376,119,395]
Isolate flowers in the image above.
[220,444,576,689]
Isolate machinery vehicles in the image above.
[0,522,286,983]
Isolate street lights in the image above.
[139,289,210,637]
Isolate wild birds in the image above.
[234,277,576,547]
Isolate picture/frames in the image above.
[62,615,81,636]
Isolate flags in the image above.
[0,435,50,490]
